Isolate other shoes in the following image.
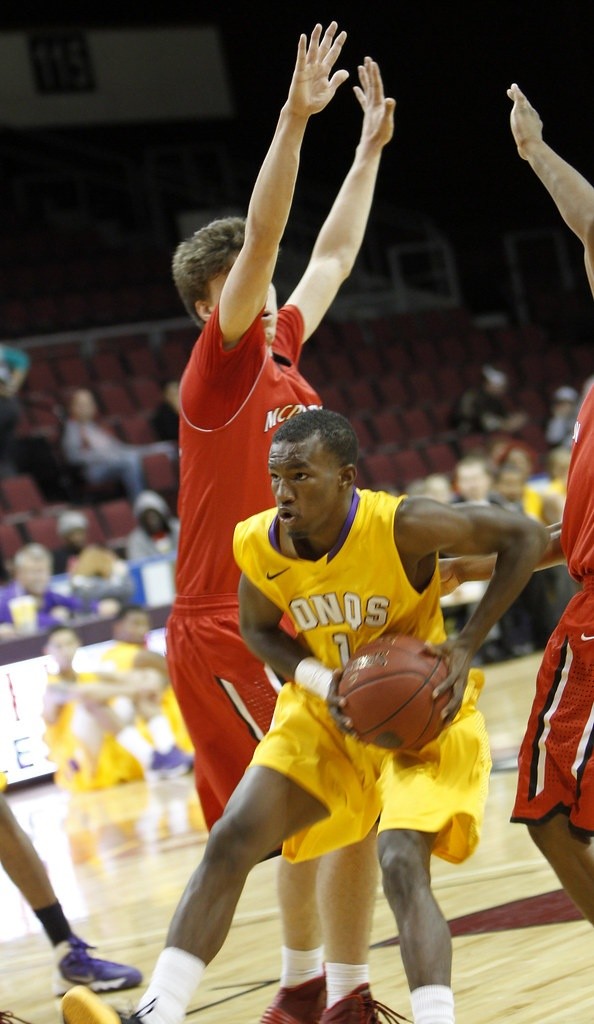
[260,972,326,1024]
[144,744,194,780]
[319,983,412,1024]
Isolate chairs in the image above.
[0,303,594,608]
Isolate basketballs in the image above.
[337,634,454,753]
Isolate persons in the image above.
[505,81,594,929]
[1,789,142,996]
[0,369,593,788]
[2,334,30,395]
[64,408,548,1024]
[165,23,397,1024]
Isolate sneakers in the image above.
[55,941,142,995]
[60,986,157,1024]
